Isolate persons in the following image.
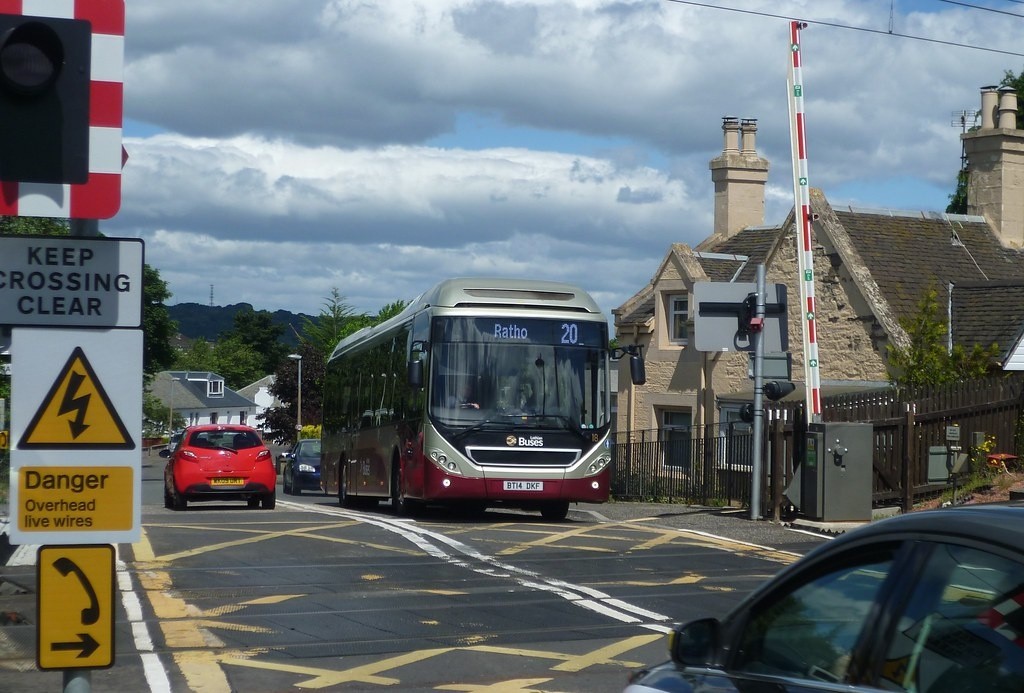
[451,382,480,409]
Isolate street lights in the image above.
[288,354,303,444]
[168,378,180,445]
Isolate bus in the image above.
[322,277,647,522]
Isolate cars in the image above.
[168,432,182,454]
[282,438,322,495]
[621,502,1024,693]
[158,422,277,511]
